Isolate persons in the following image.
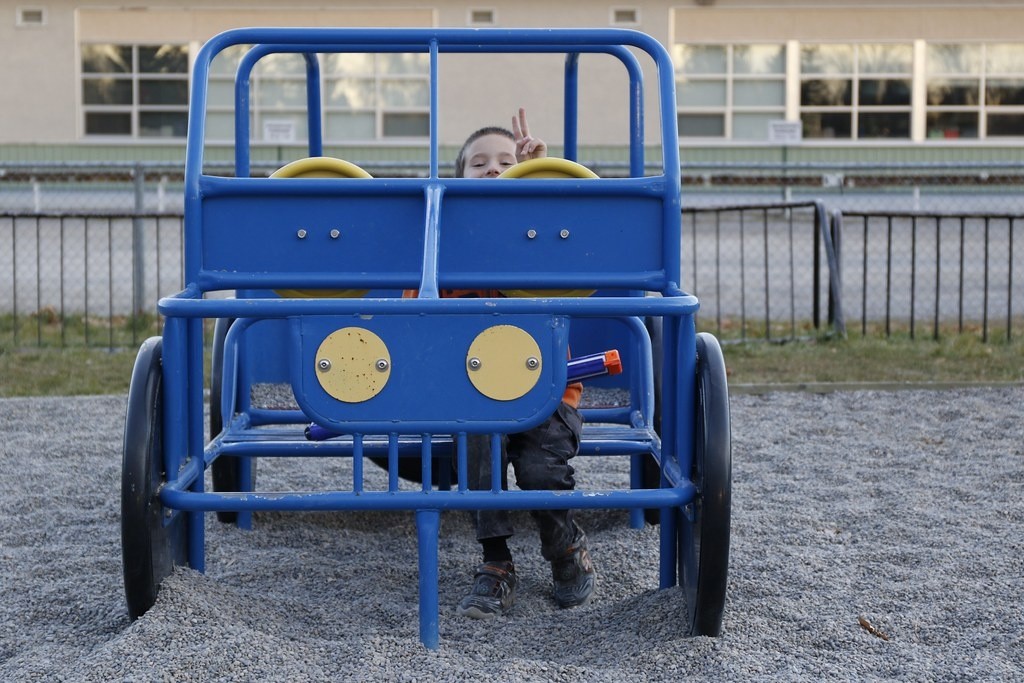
[400,111,594,619]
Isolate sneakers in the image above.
[542,523,597,607]
[456,559,520,619]
